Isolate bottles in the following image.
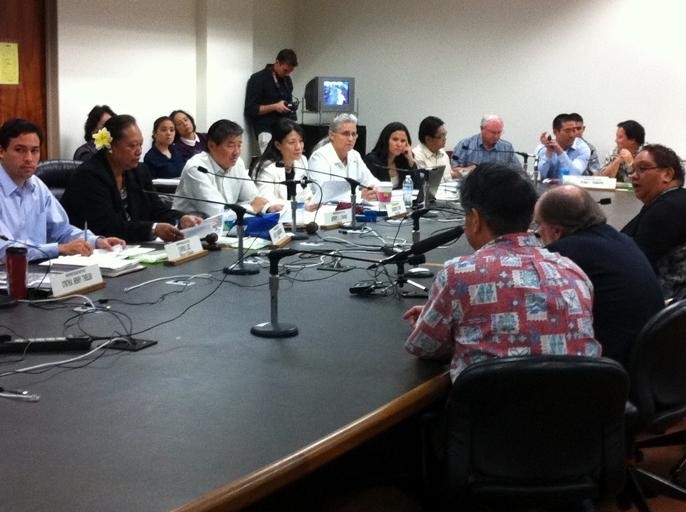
[559,159,570,185]
[295,190,306,224]
[402,175,413,208]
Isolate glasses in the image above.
[638,167,658,173]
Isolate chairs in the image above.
[616,300,686,511]
[35,159,84,200]
[422,356,630,512]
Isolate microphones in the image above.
[0,236,52,287]
[463,147,537,172]
[252,249,378,337]
[599,197,611,208]
[197,166,316,240]
[404,207,465,244]
[452,154,479,166]
[366,227,464,290]
[276,162,372,231]
[373,162,440,207]
[142,190,261,274]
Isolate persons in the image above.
[61,104,644,243]
[534,185,666,370]
[0,119,126,265]
[620,144,686,301]
[242,49,299,176]
[402,162,603,385]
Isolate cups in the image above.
[376,181,393,202]
[5,246,28,299]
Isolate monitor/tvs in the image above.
[411,164,447,203]
[307,76,354,113]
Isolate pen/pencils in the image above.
[343,231,366,234]
[85,221,87,240]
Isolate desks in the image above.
[0,181,643,512]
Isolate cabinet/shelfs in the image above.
[299,126,366,158]
